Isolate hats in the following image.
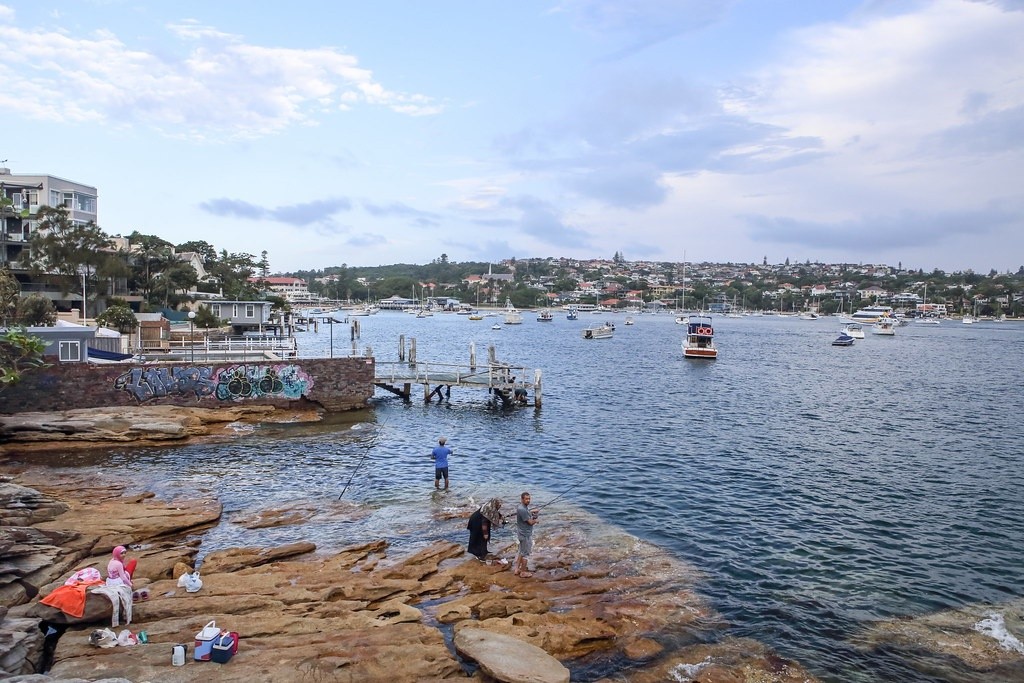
[439,438,446,444]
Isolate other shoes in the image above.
[133,592,139,600]
[141,592,147,599]
[476,555,487,562]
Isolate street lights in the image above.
[188,311,196,362]
[81,273,86,325]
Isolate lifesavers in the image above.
[705,328,712,335]
[698,328,704,335]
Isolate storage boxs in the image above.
[194,621,221,661]
[211,631,235,663]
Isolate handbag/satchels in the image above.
[177,572,203,592]
[89,628,137,648]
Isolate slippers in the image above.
[520,571,533,578]
[515,569,521,574]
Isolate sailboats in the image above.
[402,284,440,318]
[593,294,618,315]
[675,248,689,325]
[916,284,940,324]
[469,285,482,320]
[725,293,820,320]
[961,298,1003,324]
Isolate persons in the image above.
[514,492,539,578]
[466,497,505,562]
[106,546,137,589]
[431,438,453,488]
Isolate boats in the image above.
[624,316,634,325]
[831,295,906,347]
[456,308,478,315]
[537,309,553,322]
[348,289,380,316]
[582,319,617,339]
[567,307,578,320]
[681,312,718,359]
[484,296,524,330]
[309,306,338,314]
